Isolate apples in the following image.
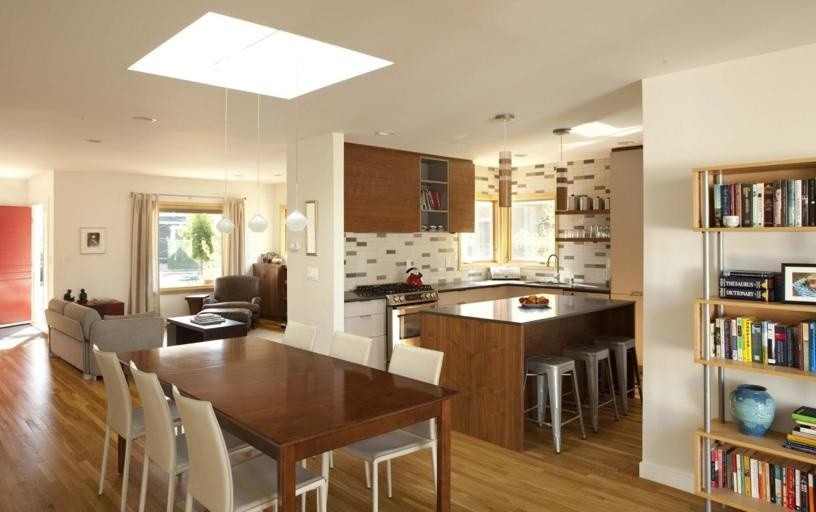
[518,295,548,304]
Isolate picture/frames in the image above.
[304,200,317,256]
[80,226,106,254]
[781,262,816,305]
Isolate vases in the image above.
[728,384,776,436]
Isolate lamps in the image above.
[495,113,572,210]
[216,60,308,234]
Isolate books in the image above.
[421,188,440,210]
[709,178,816,512]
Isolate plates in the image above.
[520,303,548,308]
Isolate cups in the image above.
[565,229,585,238]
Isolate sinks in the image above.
[525,280,569,287]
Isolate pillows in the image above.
[48,298,159,342]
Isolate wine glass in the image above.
[586,224,610,239]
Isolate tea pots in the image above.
[405,267,425,287]
[272,258,282,264]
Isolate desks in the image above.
[114,335,461,512]
[184,294,210,314]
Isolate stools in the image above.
[524,331,642,454]
[199,307,253,332]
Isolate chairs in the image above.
[203,274,262,329]
[129,363,257,510]
[44,297,165,381]
[170,384,327,511]
[93,350,183,511]
[281,321,318,351]
[312,333,374,366]
[330,344,444,512]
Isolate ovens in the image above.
[385,300,439,360]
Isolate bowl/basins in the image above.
[723,216,739,227]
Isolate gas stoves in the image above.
[356,282,440,304]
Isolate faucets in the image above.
[546,253,560,284]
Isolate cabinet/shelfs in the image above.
[690,156,816,512]
[344,142,476,233]
[253,262,286,318]
[554,209,610,242]
